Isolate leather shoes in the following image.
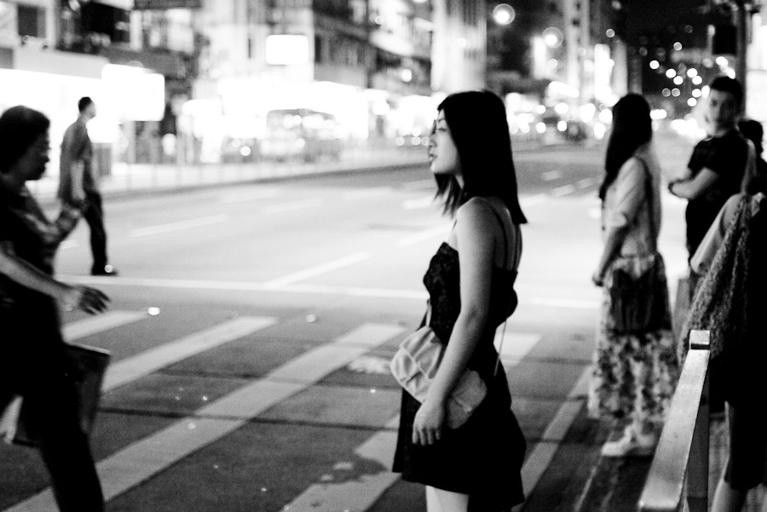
[602,428,656,457]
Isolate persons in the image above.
[736,118,766,196]
[663,76,756,305]
[690,187,766,511]
[386,88,531,511]
[48,95,121,278]
[583,93,682,459]
[0,104,115,511]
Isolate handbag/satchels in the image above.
[675,225,748,375]
[610,267,673,333]
[392,325,487,428]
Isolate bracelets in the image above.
[666,181,680,197]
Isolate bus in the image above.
[261,105,345,163]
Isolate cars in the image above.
[534,114,588,146]
[215,137,257,166]
